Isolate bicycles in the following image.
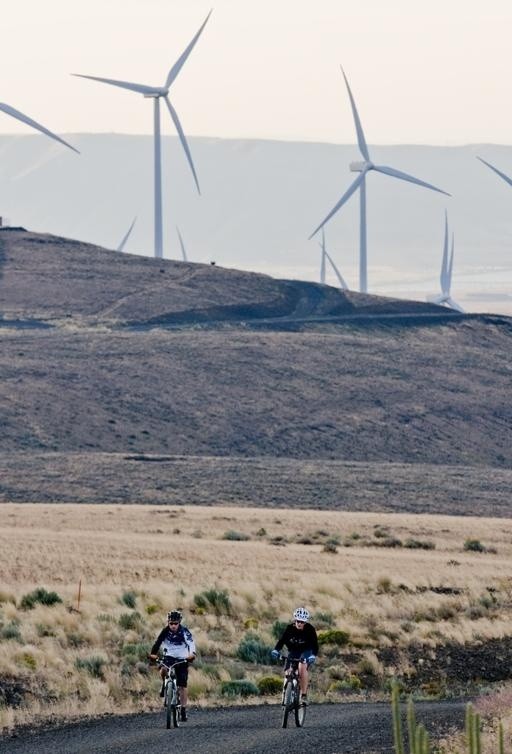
[275,653,310,729]
[146,656,195,730]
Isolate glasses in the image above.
[297,620,304,623]
[169,623,179,625]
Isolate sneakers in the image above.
[181,711,187,721]
[301,697,308,705]
[160,684,164,697]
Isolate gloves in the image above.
[307,655,315,665]
[187,656,195,663]
[150,655,157,661]
[271,650,279,658]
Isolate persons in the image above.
[269,606,319,708]
[147,609,196,723]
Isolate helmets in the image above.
[294,608,309,623]
[168,612,182,621]
